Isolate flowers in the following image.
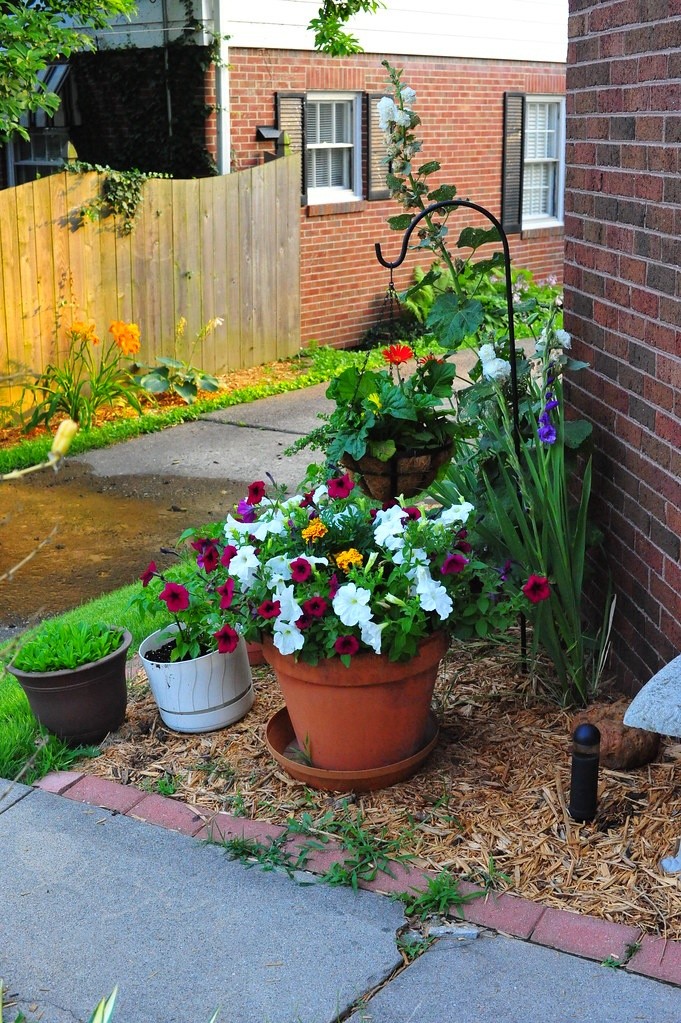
[280,341,480,468]
[137,474,554,669]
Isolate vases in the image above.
[352,437,458,503]
[259,621,451,771]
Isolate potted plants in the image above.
[5,613,133,747]
[133,566,255,735]
